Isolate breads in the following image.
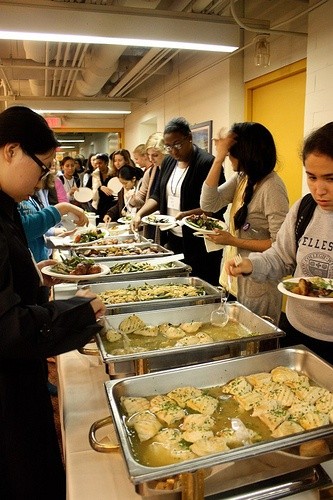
[298,439,331,457]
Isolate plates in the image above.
[117,217,132,223]
[182,214,229,235]
[74,187,94,203]
[108,225,129,231]
[140,214,177,226]
[277,276,333,303]
[40,263,111,281]
[62,228,109,246]
[107,177,123,196]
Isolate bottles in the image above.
[243,223,270,240]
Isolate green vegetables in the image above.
[51,254,96,274]
[124,281,207,301]
[77,232,105,243]
[127,376,333,466]
[154,219,165,224]
[110,314,260,354]
[188,212,223,230]
[110,261,155,273]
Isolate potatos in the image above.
[76,263,101,274]
[75,234,81,243]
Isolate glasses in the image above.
[166,139,187,151]
[26,150,56,182]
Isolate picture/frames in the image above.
[190,120,213,154]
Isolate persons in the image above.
[225,121,333,368]
[0,106,106,500]
[16,132,170,295]
[132,117,228,286]
[199,122,290,352]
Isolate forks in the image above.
[233,253,242,268]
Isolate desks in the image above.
[51,249,333,500]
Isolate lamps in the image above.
[25,100,132,115]
[0,3,239,53]
